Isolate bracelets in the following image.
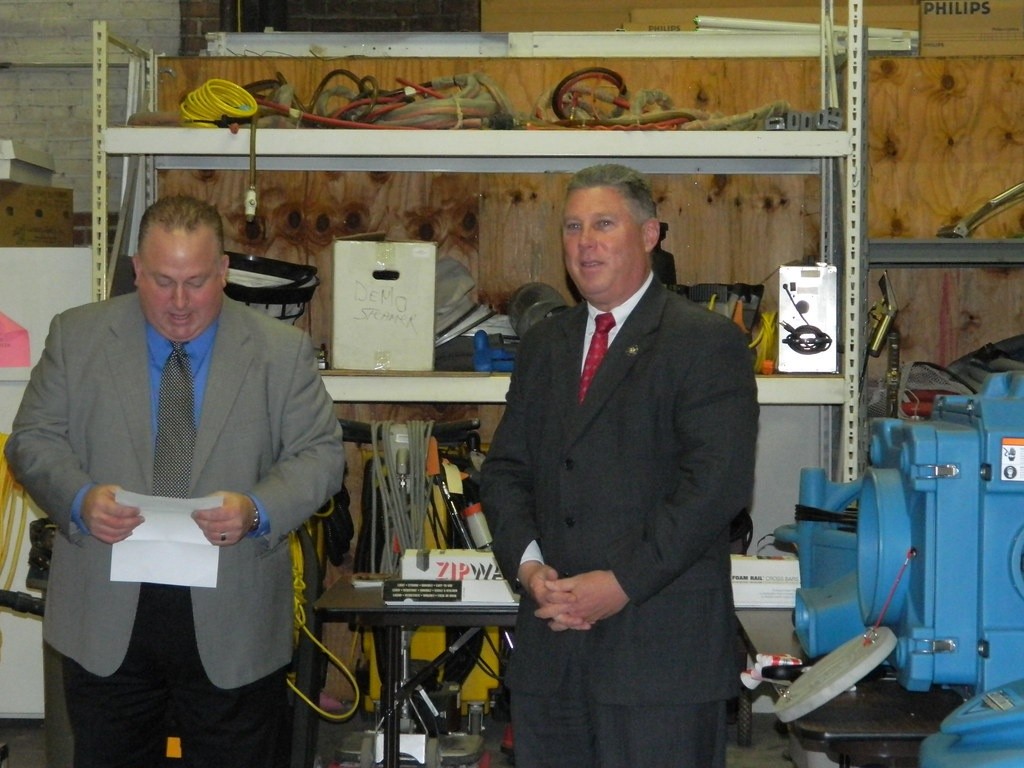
[250,508,260,532]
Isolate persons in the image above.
[4,194,346,768]
[477,165,762,768]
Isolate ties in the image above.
[578,312,617,406]
[151,339,197,499]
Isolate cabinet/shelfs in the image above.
[91,0,861,510]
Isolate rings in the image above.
[220,532,226,541]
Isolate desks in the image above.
[734,610,964,768]
[313,573,519,768]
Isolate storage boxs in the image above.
[731,554,801,609]
[0,181,74,248]
[329,230,440,372]
[919,0,1024,56]
[381,579,521,606]
[777,262,837,374]
[374,715,429,765]
[0,138,56,187]
[400,548,503,582]
[630,3,919,31]
